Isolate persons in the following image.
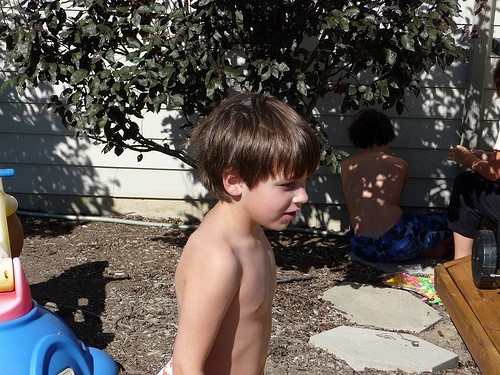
[156,95,321,374]
[341,108,453,264]
[446,58,499,260]
